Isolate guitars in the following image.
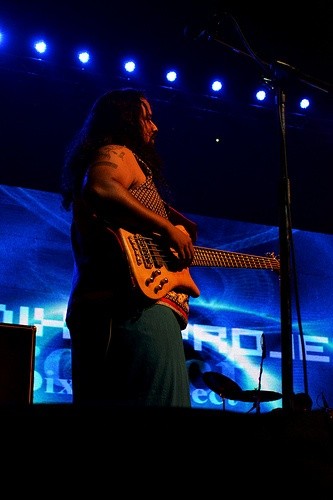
[116,226,279,299]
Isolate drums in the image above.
[237,391,282,402]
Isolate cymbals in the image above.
[202,372,242,398]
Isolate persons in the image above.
[58,89,203,500]
